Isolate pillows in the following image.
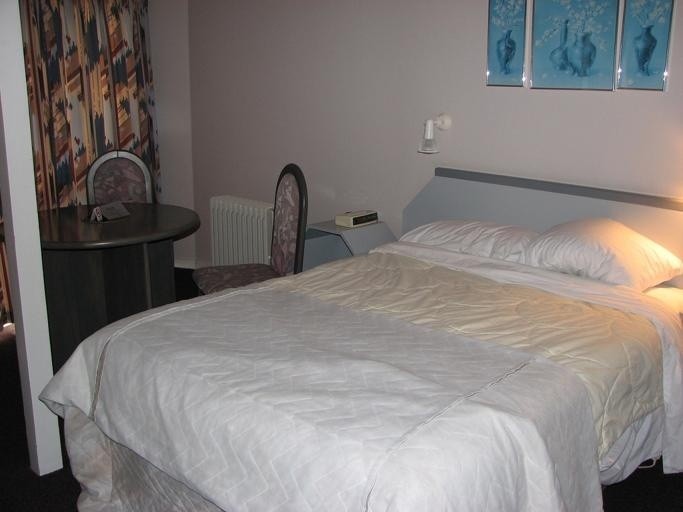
[395,219,542,266]
[522,215,682,294]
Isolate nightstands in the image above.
[305,220,395,271]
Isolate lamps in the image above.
[418,114,450,156]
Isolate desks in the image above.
[33,202,201,374]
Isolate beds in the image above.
[38,166,682,512]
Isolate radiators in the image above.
[207,194,274,267]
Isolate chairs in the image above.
[85,149,153,205]
[191,164,309,297]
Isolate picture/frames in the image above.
[485,0,675,93]
